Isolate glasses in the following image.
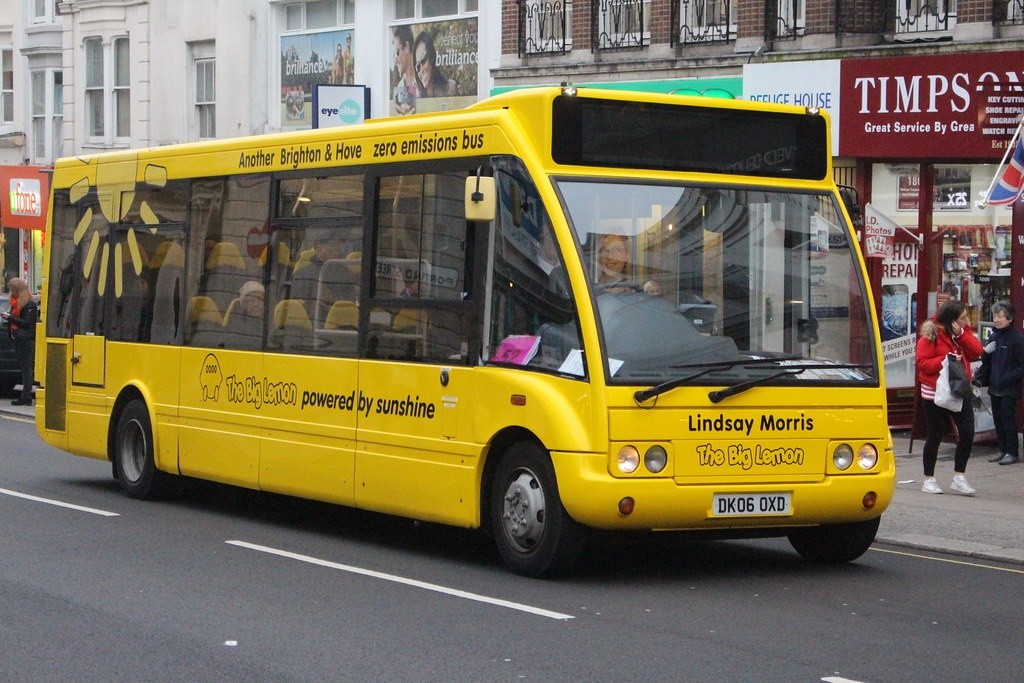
[597,245,626,258]
[537,232,553,241]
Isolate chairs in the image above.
[74,237,435,360]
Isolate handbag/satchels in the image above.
[934,352,964,413]
[947,354,973,402]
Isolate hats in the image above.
[240,281,265,309]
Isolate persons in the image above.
[0,277,39,406]
[916,300,983,496]
[101,219,609,362]
[980,300,1024,465]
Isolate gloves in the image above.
[984,341,996,354]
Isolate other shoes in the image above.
[998,453,1020,465]
[988,451,1005,462]
[11,398,32,406]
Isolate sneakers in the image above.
[921,477,943,494]
[950,475,976,494]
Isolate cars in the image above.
[0,295,38,374]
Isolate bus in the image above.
[33,81,899,580]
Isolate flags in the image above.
[865,203,895,259]
[984,122,1024,206]
